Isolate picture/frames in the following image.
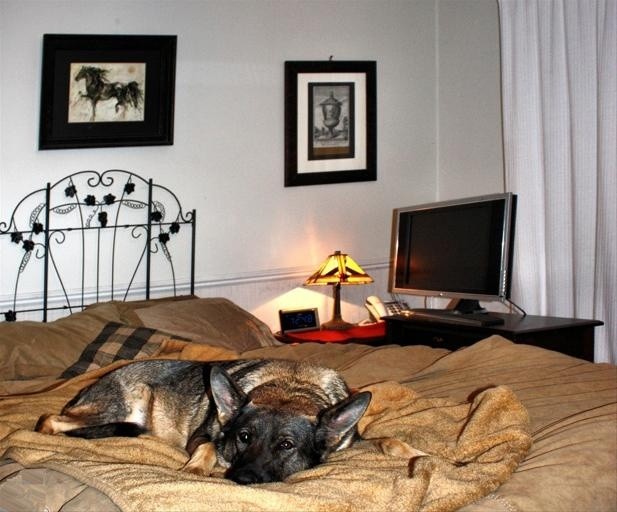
[282,61,377,188]
[36,34,179,153]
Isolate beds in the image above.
[0,169,617,512]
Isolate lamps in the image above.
[301,250,376,331]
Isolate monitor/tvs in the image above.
[388,191,517,327]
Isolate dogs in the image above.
[33,357,373,486]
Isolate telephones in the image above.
[364,295,413,322]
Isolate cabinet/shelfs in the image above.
[381,309,603,365]
